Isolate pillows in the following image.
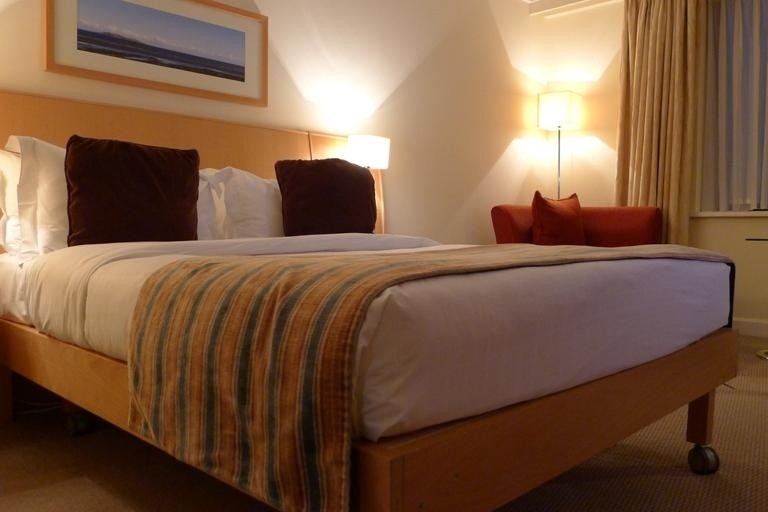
[64,135,200,248]
[275,158,377,236]
[197,168,224,240]
[1,148,21,255]
[5,134,68,256]
[207,165,283,240]
[532,190,583,244]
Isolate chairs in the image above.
[489,204,662,248]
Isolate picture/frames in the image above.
[42,0,269,107]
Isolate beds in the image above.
[0,88,739,512]
